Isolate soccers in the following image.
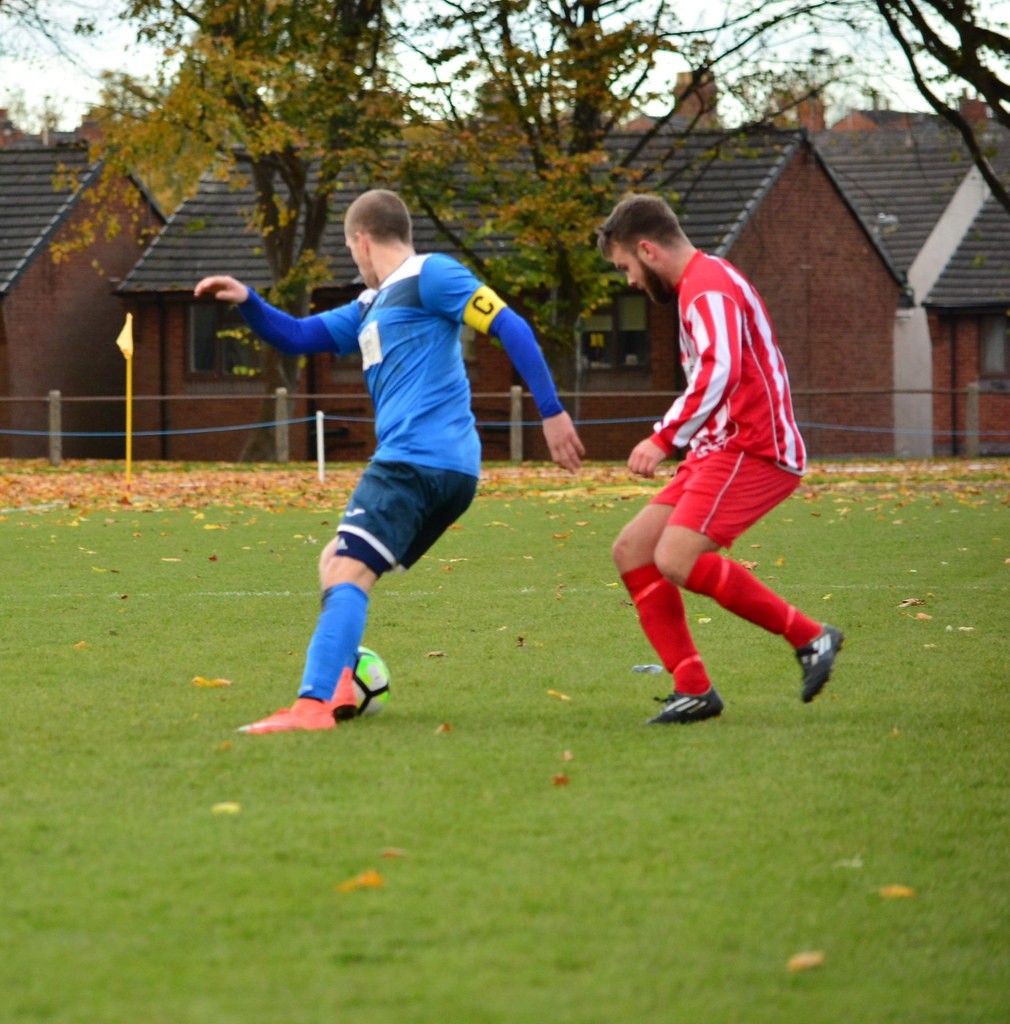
[351,646,393,722]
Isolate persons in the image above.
[598,193,844,728]
[190,186,586,734]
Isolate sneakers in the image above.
[793,618,845,704]
[237,702,336,734]
[331,668,360,723]
[644,685,725,728]
